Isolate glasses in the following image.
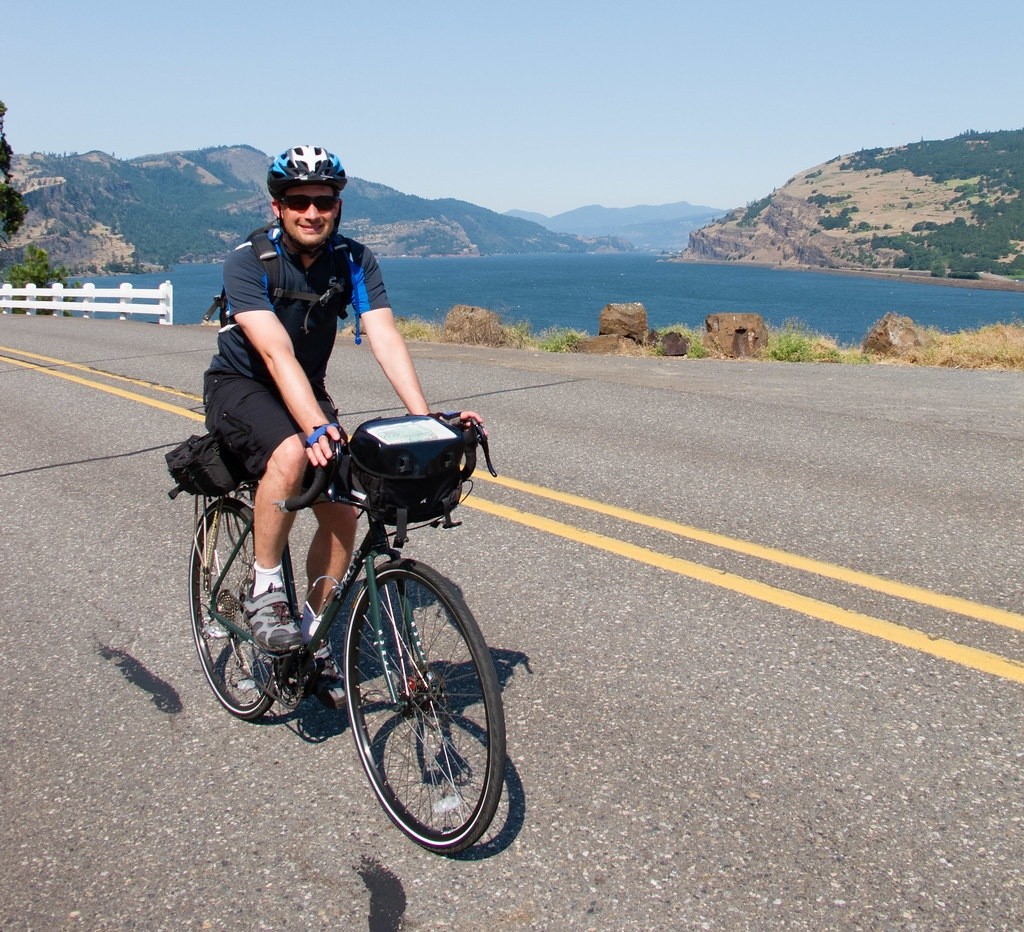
[280,194,338,211]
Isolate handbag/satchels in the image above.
[164,432,244,500]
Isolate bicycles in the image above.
[166,407,507,857]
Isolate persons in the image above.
[203,145,489,711]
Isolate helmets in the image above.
[267,144,347,199]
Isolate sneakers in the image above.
[311,640,348,710]
[242,583,302,651]
